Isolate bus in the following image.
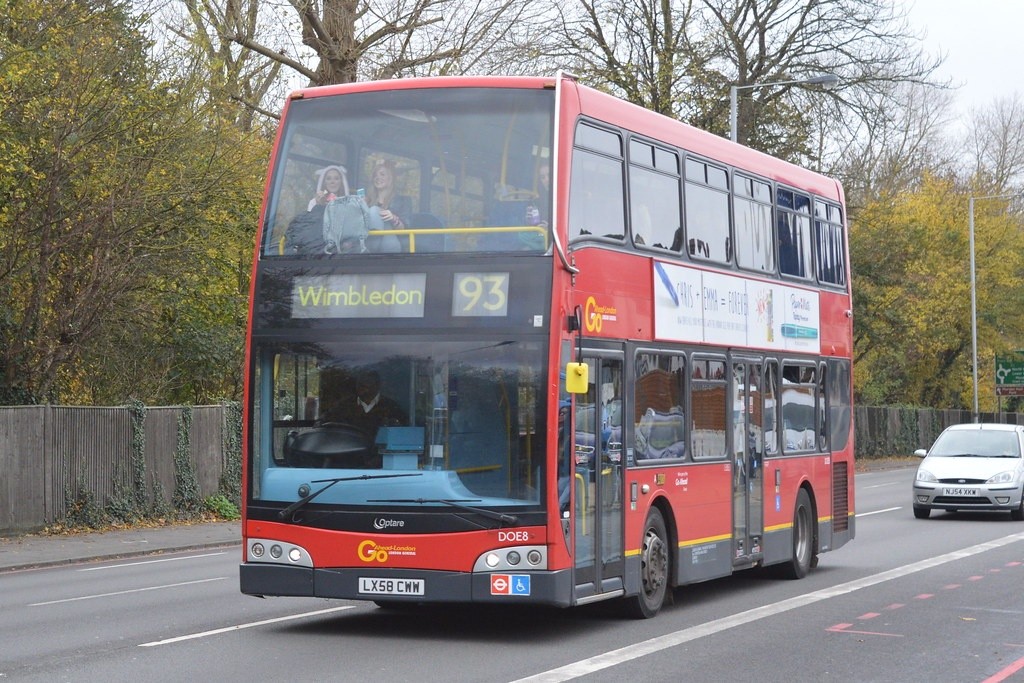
[240,69,856,619]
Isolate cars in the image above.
[912,424,1024,520]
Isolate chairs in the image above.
[633,369,827,459]
[409,214,447,253]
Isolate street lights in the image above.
[731,74,838,143]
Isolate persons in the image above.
[559,404,572,518]
[526,164,551,248]
[366,164,412,253]
[316,369,410,434]
[307,165,350,212]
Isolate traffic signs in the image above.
[995,351,1024,396]
